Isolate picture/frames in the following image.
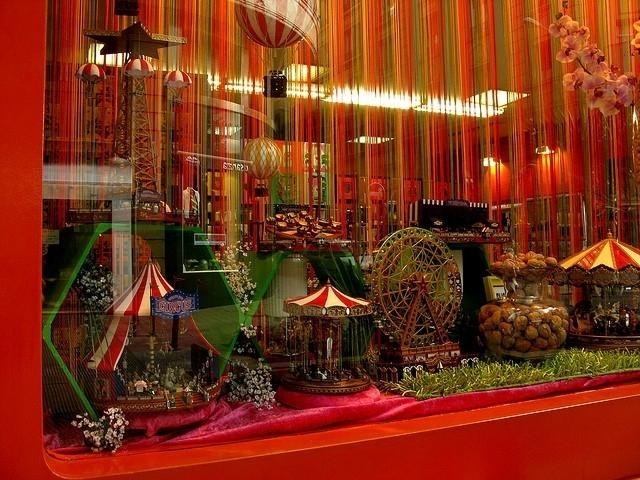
[335,174,463,208]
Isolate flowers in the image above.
[69,405,131,455]
[546,0,640,117]
[217,239,280,410]
[75,265,120,311]
